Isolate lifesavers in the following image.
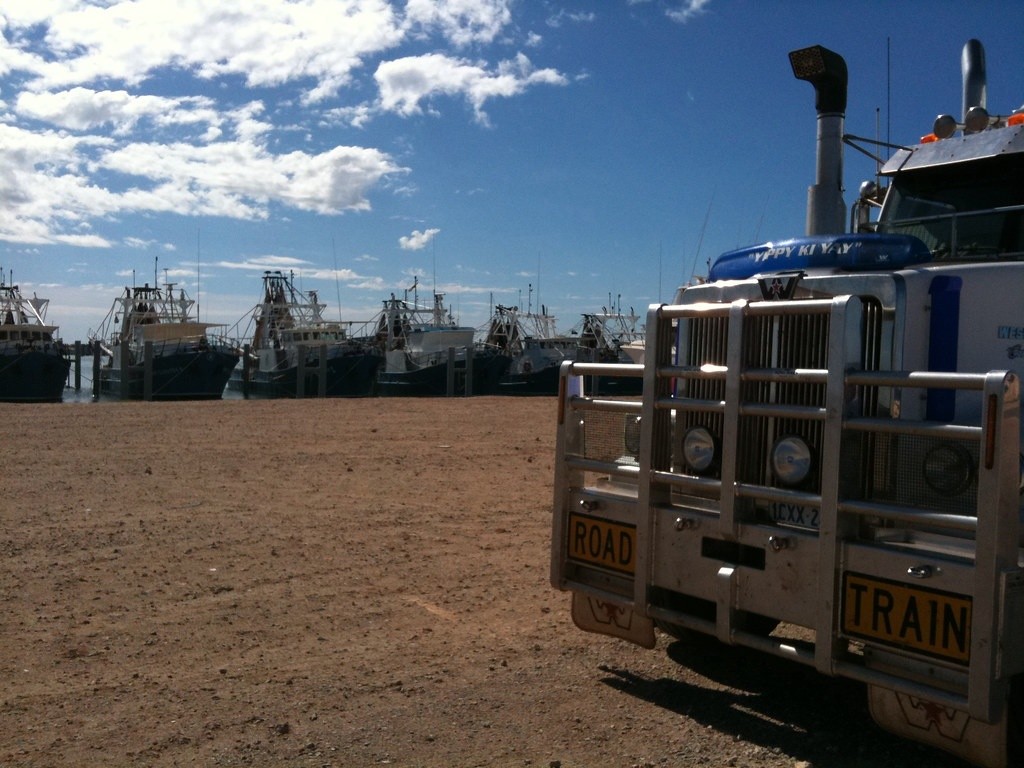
[524,361,532,372]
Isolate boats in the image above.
[0,268,73,402]
[84,256,243,400]
[236,269,643,399]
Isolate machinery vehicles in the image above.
[547,43,1024,768]
